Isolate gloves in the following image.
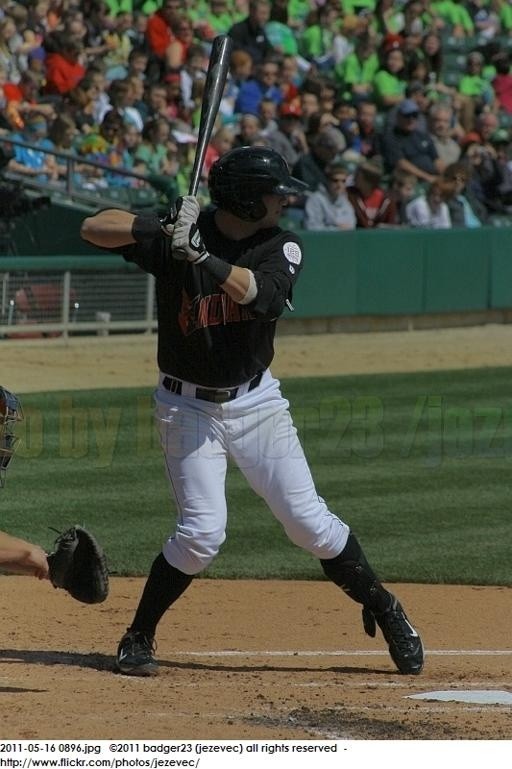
[159,195,210,264]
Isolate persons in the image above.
[0,525,109,604]
[80,146,424,676]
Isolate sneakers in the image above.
[375,593,423,674]
[116,628,158,676]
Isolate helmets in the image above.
[0,385,24,473]
[208,146,310,222]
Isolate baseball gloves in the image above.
[46,524,109,604]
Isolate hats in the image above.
[400,98,419,115]
[279,101,303,119]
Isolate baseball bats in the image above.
[171,35,237,259]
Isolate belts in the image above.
[163,373,262,402]
